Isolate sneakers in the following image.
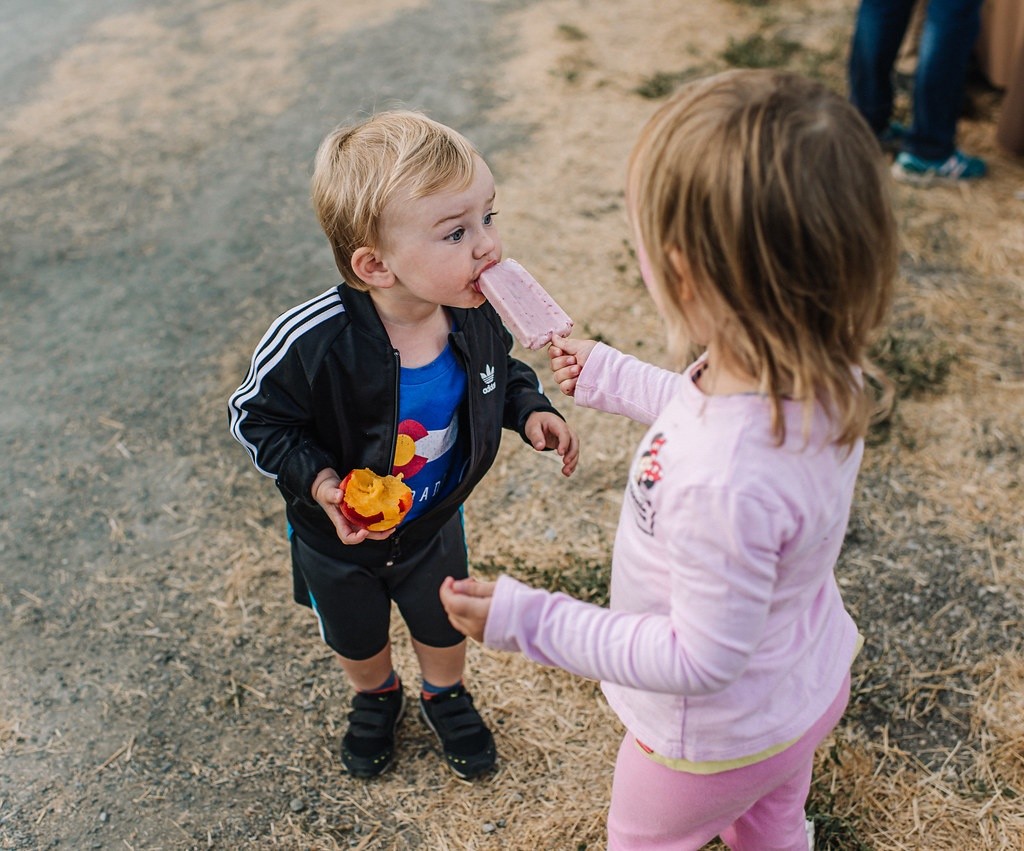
[418,682,496,779]
[883,122,916,155]
[890,149,993,190]
[338,673,407,779]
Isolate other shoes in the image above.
[964,103,994,124]
[979,82,1007,107]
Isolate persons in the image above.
[223,112,584,781]
[439,65,905,851]
[845,0,990,177]
